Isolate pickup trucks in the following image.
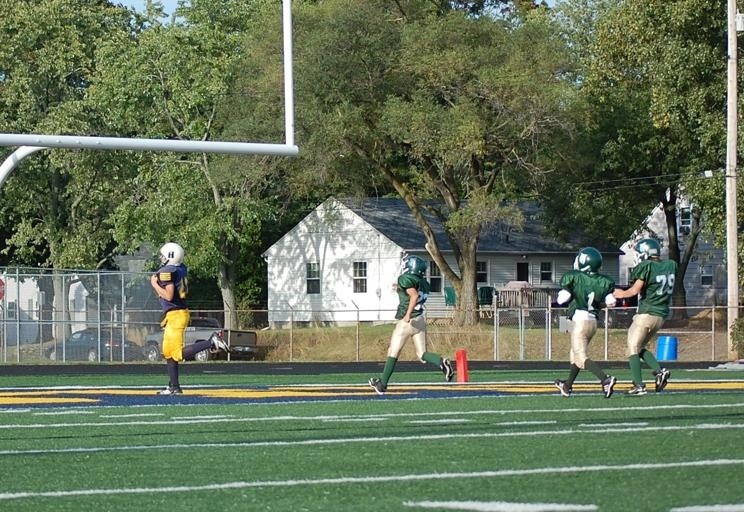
[140,315,256,362]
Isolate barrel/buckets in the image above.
[655,335,678,361]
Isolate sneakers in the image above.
[157,385,183,396]
[209,333,231,353]
[440,358,455,382]
[368,377,386,396]
[625,384,648,397]
[554,378,572,398]
[602,374,618,398]
[656,368,671,391]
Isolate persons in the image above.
[148,242,191,396]
[554,246,616,399]
[612,238,678,397]
[368,255,454,396]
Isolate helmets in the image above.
[160,243,185,266]
[400,254,427,277]
[574,247,603,272]
[633,239,661,261]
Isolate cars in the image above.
[44,327,140,363]
[548,285,638,328]
